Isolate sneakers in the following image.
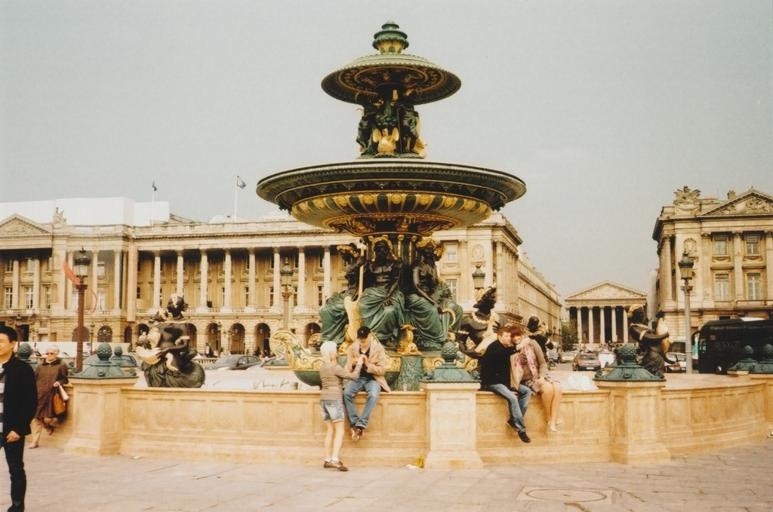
[350,426,363,441]
[517,430,531,443]
[545,418,563,432]
[324,458,348,472]
[27,443,40,449]
[506,419,519,432]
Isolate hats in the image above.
[356,325,371,339]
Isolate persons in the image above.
[140,296,205,388]
[315,237,462,351]
[317,341,364,473]
[458,286,510,358]
[0,326,38,512]
[509,326,562,432]
[205,342,219,363]
[344,326,387,442]
[478,326,532,443]
[27,345,69,450]
[628,304,674,376]
[245,346,268,360]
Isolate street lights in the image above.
[278,256,294,331]
[471,264,486,298]
[677,248,695,375]
[88,323,96,355]
[72,245,89,372]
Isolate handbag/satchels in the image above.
[53,390,66,415]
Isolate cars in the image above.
[548,350,578,363]
[572,351,600,371]
[599,349,617,369]
[204,354,263,370]
[664,352,687,372]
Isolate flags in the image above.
[237,177,246,190]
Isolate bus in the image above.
[690,318,772,374]
